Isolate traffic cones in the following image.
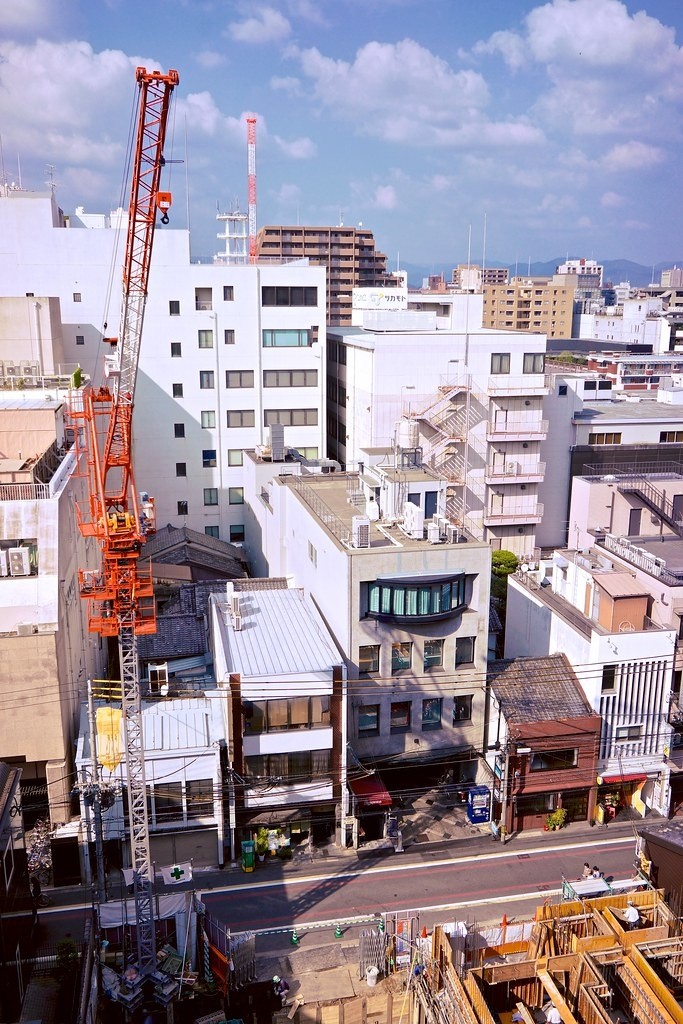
[291,927,300,943]
[501,913,508,926]
[379,919,384,931]
[421,926,428,938]
[335,922,345,937]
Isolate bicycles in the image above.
[437,780,451,800]
[28,813,52,905]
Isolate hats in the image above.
[551,1000,556,1006]
[627,901,633,905]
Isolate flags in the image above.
[160,862,192,884]
[123,866,154,886]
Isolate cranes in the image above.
[69,68,181,1004]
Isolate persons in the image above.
[583,862,599,880]
[412,966,425,981]
[273,975,290,1001]
[546,1002,563,1024]
[624,901,639,929]
